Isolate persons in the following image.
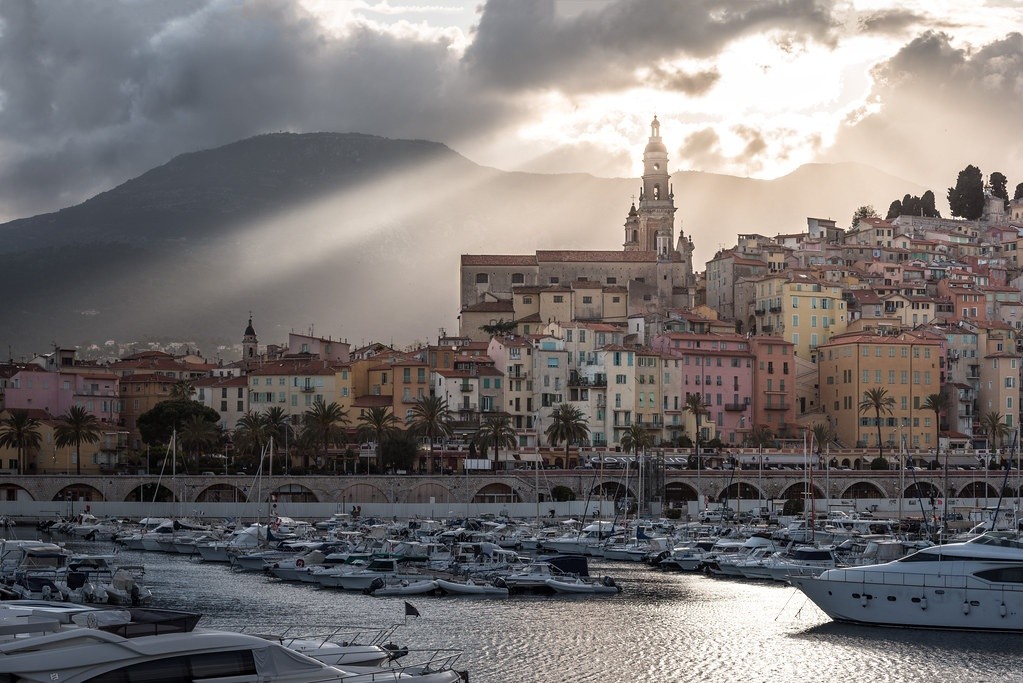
[282,464,290,474]
[400,580,407,587]
[131,584,139,606]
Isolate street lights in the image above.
[583,415,592,470]
[283,421,288,475]
[889,429,896,470]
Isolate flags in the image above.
[404,601,421,618]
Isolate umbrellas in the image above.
[202,453,228,458]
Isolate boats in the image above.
[0,515,468,683]
[37,429,1023,597]
[781,422,1023,633]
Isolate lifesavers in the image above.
[296,559,304,567]
